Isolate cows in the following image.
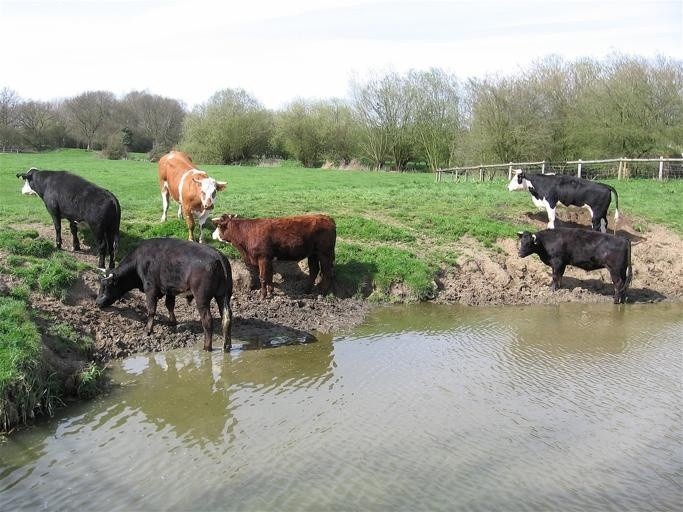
[158,150,228,245]
[210,213,337,301]
[506,168,619,236]
[95,237,233,353]
[513,227,633,305]
[16,166,121,269]
